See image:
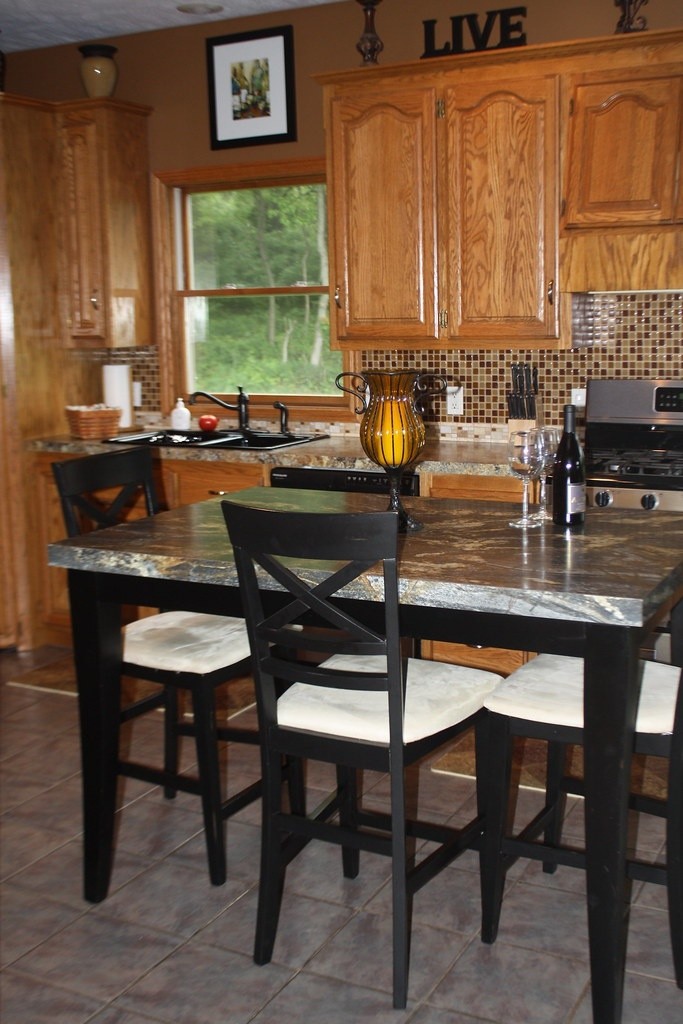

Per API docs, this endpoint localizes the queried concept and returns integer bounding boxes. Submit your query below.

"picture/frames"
[202,23,297,150]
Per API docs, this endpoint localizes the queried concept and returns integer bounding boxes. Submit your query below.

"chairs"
[220,500,507,1010]
[481,563,682,1024]
[271,462,420,496]
[50,445,261,904]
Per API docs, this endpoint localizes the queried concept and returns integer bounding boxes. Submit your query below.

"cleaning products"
[171,398,191,430]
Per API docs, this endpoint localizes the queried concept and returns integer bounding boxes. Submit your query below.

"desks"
[47,476,683,1023]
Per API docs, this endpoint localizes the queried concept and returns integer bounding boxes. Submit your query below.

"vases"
[80,44,120,101]
[356,2,383,67]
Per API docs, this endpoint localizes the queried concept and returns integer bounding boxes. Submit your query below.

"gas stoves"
[585,379,683,511]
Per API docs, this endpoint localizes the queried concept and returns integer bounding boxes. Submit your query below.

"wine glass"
[528,427,558,520]
[508,432,546,528]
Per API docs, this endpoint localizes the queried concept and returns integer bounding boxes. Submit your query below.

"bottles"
[170,398,191,442]
[553,405,585,526]
[78,44,119,97]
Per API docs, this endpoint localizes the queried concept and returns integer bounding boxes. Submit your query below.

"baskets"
[65,405,122,439]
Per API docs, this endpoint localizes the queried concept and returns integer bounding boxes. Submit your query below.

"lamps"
[334,365,462,536]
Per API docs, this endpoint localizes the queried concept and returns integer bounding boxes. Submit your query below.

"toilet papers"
[104,365,131,428]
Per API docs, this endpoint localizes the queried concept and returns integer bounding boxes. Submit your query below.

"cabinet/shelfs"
[51,102,154,353]
[311,29,683,349]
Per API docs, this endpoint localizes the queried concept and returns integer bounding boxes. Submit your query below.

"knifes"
[505,364,538,420]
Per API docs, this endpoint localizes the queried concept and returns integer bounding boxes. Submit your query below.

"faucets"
[273,401,288,433]
[190,386,251,430]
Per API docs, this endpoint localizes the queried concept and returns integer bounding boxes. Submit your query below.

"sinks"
[101,430,230,448]
[208,437,310,450]
